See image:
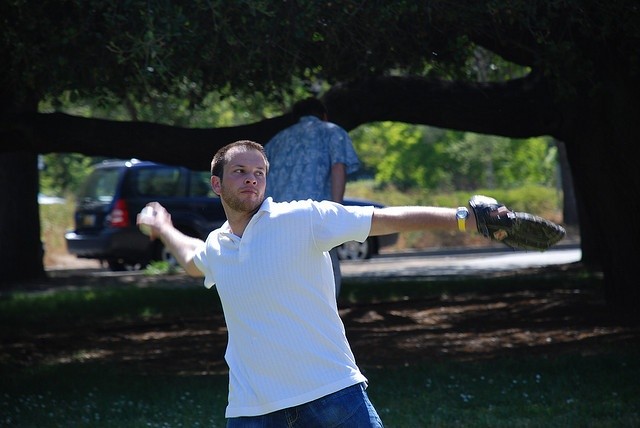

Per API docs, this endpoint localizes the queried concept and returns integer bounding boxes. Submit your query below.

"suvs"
[63,160,400,273]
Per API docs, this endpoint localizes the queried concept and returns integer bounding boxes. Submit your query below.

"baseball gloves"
[471,195,567,254]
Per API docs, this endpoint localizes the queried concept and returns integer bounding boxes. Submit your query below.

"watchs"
[456,206,469,232]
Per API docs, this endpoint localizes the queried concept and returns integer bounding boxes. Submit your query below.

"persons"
[137,140,567,428]
[263,97,360,303]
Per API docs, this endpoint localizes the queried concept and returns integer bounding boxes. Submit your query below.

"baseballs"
[137,204,158,236]
